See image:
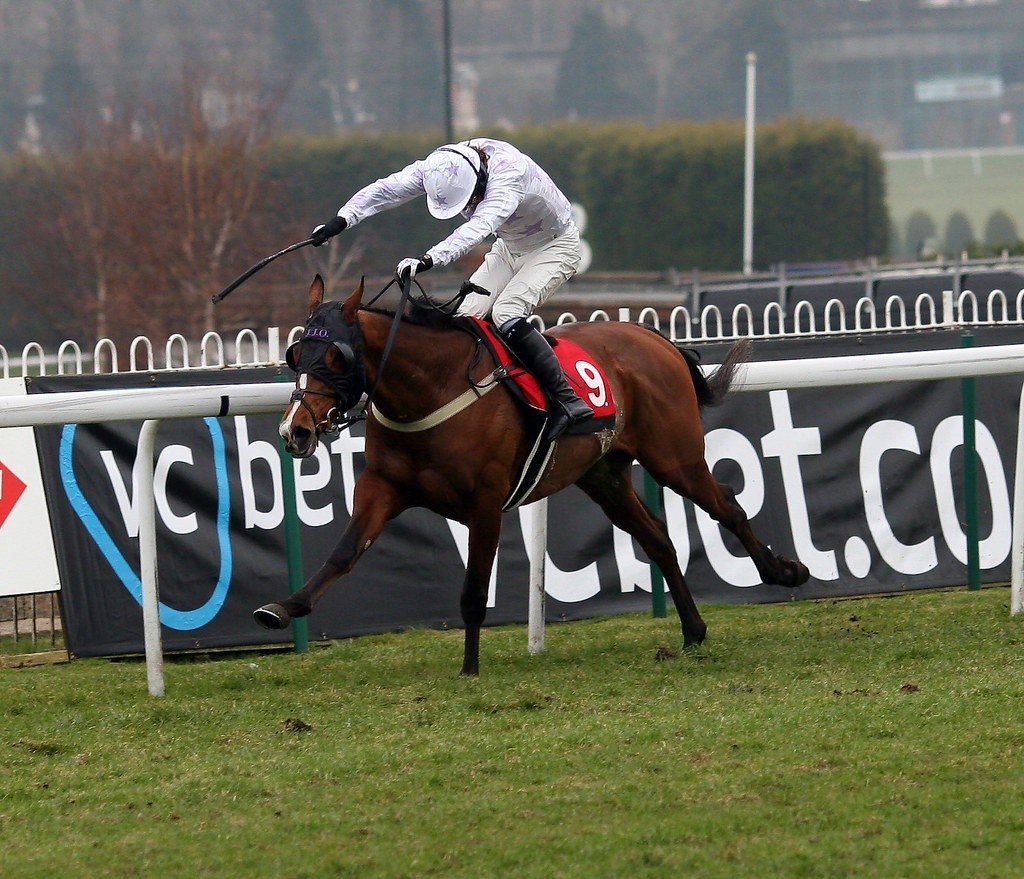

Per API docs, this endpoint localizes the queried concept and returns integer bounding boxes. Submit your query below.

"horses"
[255,272,810,674]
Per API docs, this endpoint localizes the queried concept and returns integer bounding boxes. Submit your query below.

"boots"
[512,328,594,440]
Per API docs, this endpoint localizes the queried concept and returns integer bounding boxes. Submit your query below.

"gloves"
[396,253,433,281]
[309,216,347,247]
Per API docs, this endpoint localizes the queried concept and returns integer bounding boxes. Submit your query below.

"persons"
[310,138,595,441]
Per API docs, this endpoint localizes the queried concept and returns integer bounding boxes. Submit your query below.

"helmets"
[422,143,481,219]
